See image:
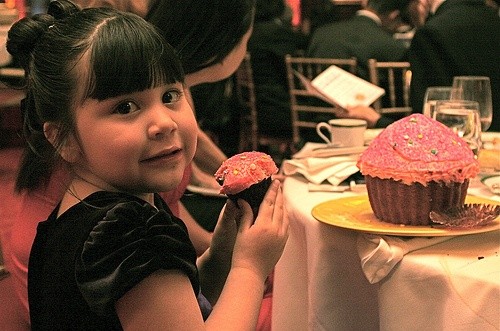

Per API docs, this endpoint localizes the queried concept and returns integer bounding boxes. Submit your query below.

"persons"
[246,0,310,138]
[141,0,287,260]
[5,6,291,330]
[307,0,409,144]
[332,0,500,135]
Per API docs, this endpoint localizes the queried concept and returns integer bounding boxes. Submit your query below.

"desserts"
[357,113,479,225]
[214,151,279,208]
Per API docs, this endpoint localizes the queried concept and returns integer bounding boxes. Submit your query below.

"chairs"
[369,58,413,117]
[285,54,356,146]
[235,52,290,164]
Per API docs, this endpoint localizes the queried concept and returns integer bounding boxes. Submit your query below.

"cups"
[422,87,464,121]
[435,99,482,160]
[316,118,368,148]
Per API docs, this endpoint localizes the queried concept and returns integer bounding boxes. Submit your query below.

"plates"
[310,193,500,236]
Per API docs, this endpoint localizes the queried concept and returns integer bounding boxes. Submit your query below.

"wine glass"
[449,76,493,159]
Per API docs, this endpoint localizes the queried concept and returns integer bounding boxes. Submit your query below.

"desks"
[270,132,500,330]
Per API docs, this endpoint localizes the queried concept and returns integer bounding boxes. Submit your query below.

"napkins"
[357,233,454,286]
[280,157,361,186]
